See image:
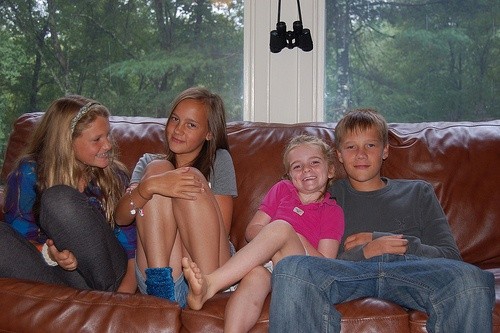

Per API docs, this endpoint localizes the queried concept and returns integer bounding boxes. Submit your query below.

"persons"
[269,107,496,333]
[181,135,345,333]
[0,94,138,294]
[114,85,240,308]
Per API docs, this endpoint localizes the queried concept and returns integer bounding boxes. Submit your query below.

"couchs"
[0,112,500,333]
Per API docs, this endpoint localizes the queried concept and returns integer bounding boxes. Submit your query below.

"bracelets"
[126,190,145,216]
[41,242,60,267]
[136,182,153,201]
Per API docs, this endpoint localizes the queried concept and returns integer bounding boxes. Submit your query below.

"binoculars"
[269,21,314,53]
